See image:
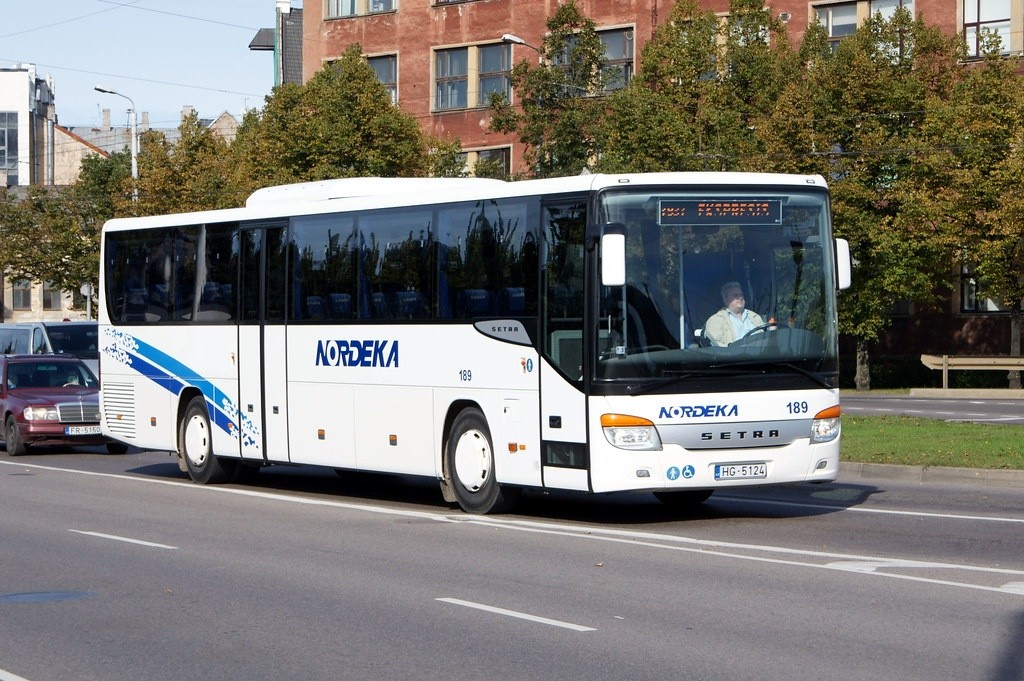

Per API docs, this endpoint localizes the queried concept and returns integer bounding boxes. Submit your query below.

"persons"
[704,281,776,347]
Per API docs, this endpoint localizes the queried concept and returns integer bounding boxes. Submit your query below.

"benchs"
[921,353,1024,388]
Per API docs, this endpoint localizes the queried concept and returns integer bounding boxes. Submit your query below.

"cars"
[0,355,129,457]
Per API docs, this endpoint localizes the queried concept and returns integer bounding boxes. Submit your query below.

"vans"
[0,323,103,387]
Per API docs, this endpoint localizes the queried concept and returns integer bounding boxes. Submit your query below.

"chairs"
[115,281,526,321]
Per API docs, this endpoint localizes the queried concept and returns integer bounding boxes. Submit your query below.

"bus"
[98,179,853,515]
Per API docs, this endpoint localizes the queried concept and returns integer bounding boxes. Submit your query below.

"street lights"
[91,85,141,217]
[501,34,543,176]
